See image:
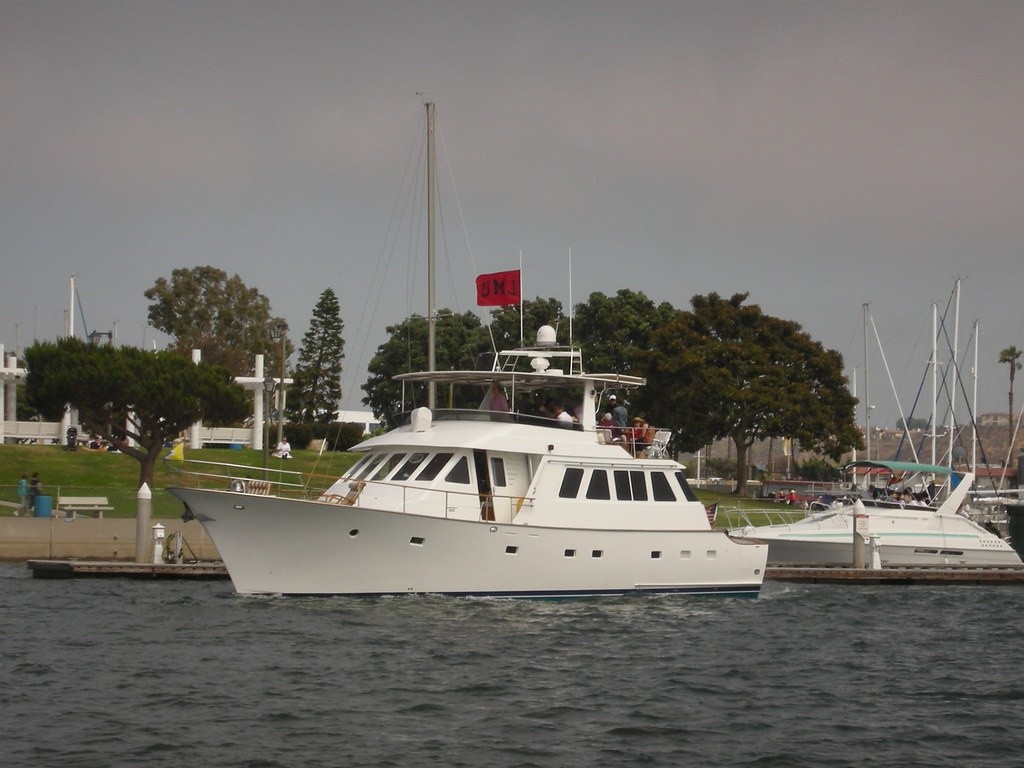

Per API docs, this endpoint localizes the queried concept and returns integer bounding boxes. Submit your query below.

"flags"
[704,502,719,524]
[476,270,521,307]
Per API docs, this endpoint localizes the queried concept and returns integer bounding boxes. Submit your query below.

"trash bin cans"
[33,493,52,517]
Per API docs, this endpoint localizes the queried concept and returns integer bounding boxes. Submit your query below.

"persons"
[14,472,45,516]
[490,381,656,460]
[271,437,293,458]
[84,435,127,453]
[770,480,1001,538]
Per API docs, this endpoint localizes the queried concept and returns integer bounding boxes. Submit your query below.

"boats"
[161,92,770,595]
[722,458,1024,570]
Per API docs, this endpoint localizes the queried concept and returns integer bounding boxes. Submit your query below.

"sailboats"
[813,277,1024,538]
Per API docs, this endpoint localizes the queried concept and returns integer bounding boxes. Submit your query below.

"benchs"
[59,496,114,519]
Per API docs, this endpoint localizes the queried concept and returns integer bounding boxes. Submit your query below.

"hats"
[609,394,617,401]
[790,490,796,493]
[633,417,644,422]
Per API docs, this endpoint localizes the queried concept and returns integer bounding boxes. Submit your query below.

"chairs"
[646,432,672,459]
